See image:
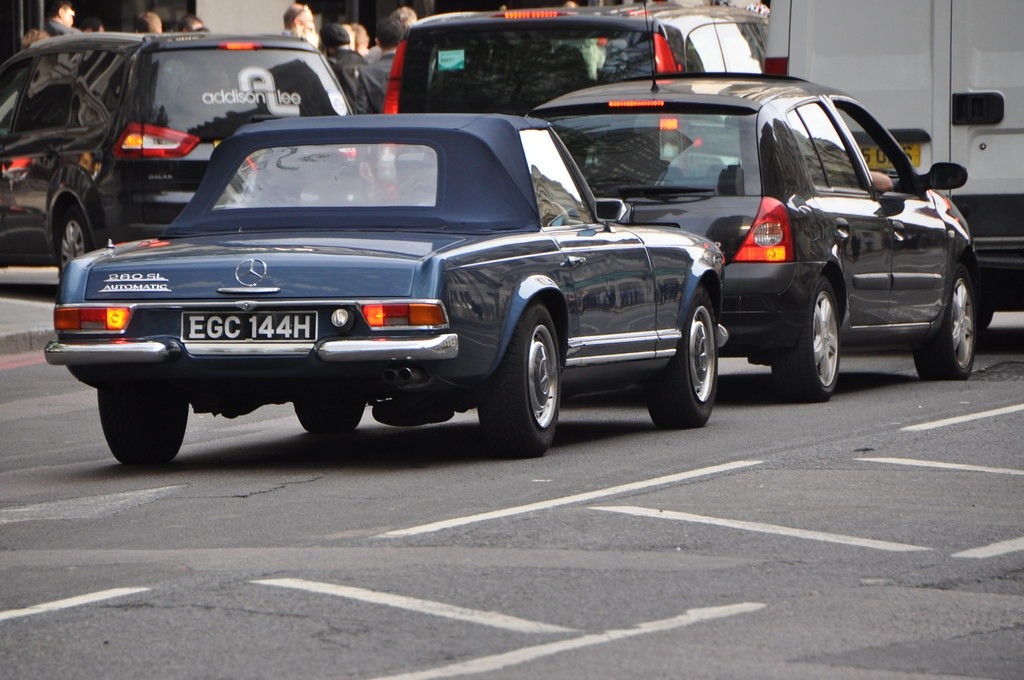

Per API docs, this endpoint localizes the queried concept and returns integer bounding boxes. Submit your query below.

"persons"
[869,171,892,192]
[281,2,418,115]
[20,0,210,49]
[544,0,606,83]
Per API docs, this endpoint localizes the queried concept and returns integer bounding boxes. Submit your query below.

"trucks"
[764,1,1024,325]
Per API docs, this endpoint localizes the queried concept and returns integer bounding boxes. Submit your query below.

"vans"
[385,1,769,157]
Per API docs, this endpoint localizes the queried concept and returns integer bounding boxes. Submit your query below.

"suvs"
[0,31,367,284]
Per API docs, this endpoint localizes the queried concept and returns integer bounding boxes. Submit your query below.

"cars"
[520,72,987,403]
[44,112,728,466]
